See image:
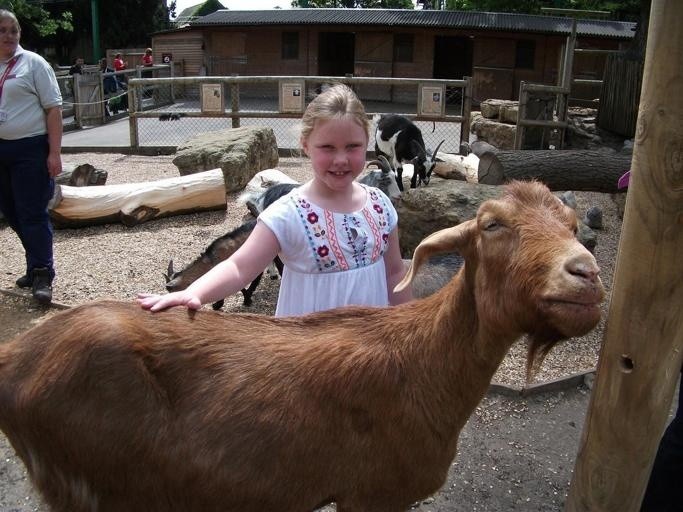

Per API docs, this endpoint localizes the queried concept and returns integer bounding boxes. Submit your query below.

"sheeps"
[162,221,265,311]
[234,169,302,281]
[256,155,403,285]
[372,111,446,186]
[1,176,607,512]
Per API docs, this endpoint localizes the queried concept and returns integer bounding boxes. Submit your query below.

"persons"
[66,58,85,120]
[0,9,64,301]
[97,53,127,116]
[142,48,153,98]
[135,84,414,311]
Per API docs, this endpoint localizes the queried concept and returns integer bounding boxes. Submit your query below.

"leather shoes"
[32,266,53,303]
[16,272,32,287]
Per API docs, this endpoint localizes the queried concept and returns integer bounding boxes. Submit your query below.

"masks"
[47,176,62,215]
